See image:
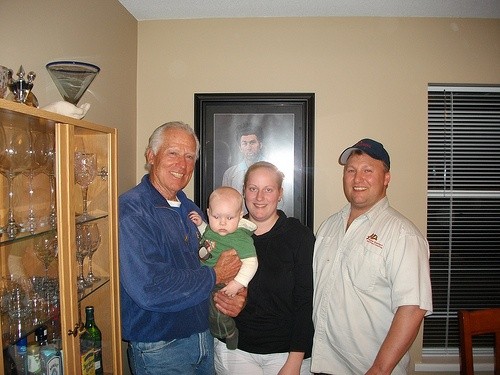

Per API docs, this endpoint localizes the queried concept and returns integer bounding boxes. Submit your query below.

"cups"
[0,274,60,321]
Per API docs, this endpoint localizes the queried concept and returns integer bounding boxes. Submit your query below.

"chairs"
[457,309,500,375]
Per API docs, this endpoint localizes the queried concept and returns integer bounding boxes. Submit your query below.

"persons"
[214,161,316,375]
[188,187,258,350]
[118,121,247,375]
[310,139,433,375]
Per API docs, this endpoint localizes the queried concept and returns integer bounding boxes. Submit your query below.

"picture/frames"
[194,93,315,232]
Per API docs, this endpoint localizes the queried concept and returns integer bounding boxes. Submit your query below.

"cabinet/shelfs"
[0,98,123,375]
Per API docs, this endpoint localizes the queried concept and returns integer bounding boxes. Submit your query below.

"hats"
[338,138,391,166]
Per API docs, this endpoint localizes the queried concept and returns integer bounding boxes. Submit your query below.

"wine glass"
[75,224,93,292]
[46,60,101,106]
[20,129,50,231]
[0,122,31,234]
[43,132,57,226]
[84,223,101,282]
[34,232,58,276]
[74,153,97,221]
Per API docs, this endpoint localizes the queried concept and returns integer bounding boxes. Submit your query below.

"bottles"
[4,320,64,375]
[85,306,103,375]
